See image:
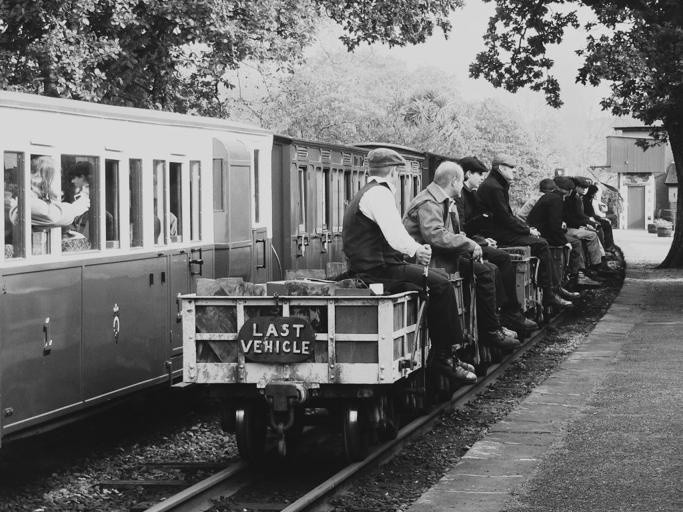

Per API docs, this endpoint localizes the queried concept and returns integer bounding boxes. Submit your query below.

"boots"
[428,346,477,384]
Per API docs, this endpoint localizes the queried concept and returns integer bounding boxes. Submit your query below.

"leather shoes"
[571,271,600,288]
[503,311,538,331]
[542,287,580,307]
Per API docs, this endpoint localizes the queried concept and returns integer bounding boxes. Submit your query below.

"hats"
[460,157,488,173]
[555,176,590,191]
[368,148,406,167]
[493,154,517,168]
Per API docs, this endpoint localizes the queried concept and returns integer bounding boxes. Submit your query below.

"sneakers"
[482,325,520,345]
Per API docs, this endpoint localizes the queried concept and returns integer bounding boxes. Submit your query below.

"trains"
[0,91,461,451]
[163,220,599,466]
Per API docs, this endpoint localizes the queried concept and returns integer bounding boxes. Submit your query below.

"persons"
[478,158,580,308]
[340,146,478,386]
[516,177,617,289]
[455,158,539,332]
[398,159,521,347]
[4,156,177,244]
